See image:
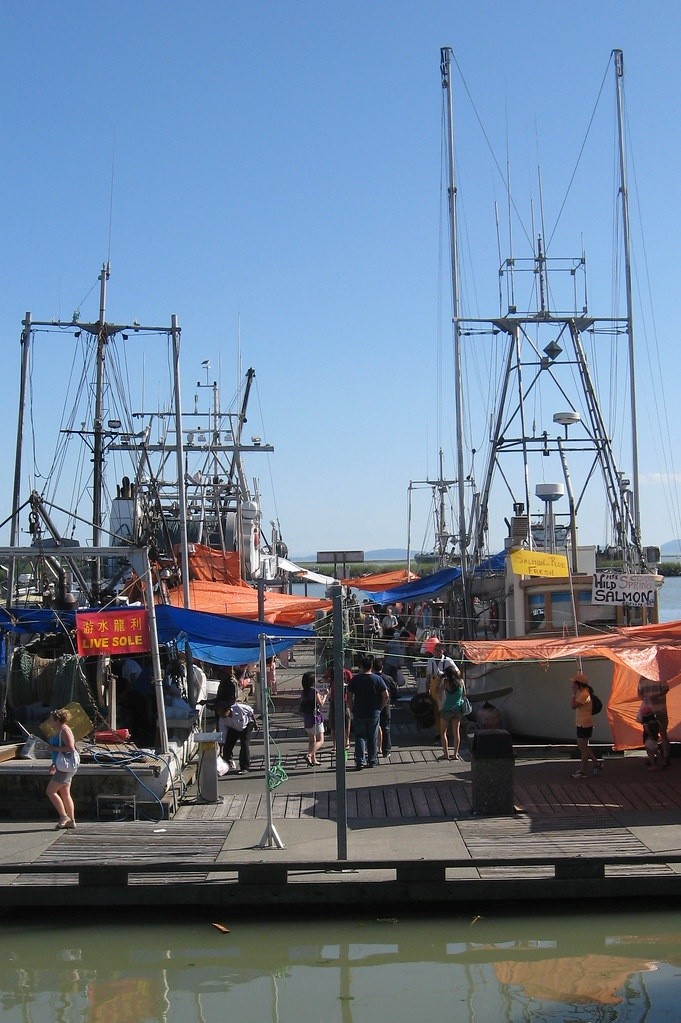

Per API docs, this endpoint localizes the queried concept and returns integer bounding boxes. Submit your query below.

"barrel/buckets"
[409,693,435,729]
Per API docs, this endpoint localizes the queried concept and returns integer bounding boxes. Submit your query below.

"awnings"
[0,543,509,673]
[460,621,681,752]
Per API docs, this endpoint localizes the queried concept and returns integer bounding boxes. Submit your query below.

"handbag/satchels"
[461,699,472,715]
[396,669,406,687]
[55,746,81,773]
[591,694,603,715]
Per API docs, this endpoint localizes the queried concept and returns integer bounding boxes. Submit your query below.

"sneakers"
[592,761,605,777]
[568,767,592,779]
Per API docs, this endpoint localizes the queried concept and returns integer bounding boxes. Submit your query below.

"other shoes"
[303,755,314,768]
[68,819,77,829]
[56,815,71,829]
[314,760,323,766]
[438,754,449,760]
[332,745,391,758]
[368,762,376,768]
[449,755,459,760]
[356,765,364,770]
[241,769,248,775]
[647,758,670,770]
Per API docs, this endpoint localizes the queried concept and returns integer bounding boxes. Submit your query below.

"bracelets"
[571,694,576,696]
[572,691,576,694]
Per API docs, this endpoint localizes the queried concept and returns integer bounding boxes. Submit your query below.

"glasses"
[50,710,57,714]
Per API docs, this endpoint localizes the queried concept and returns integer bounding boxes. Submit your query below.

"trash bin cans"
[470,729,518,818]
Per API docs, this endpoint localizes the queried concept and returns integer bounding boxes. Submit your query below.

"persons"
[635,676,671,771]
[350,592,498,760]
[299,671,330,767]
[568,675,605,779]
[117,658,154,747]
[321,658,398,770]
[199,665,258,775]
[45,709,81,829]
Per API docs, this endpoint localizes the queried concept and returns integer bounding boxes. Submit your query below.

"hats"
[440,666,457,676]
[569,674,588,684]
[362,598,368,603]
[640,706,653,716]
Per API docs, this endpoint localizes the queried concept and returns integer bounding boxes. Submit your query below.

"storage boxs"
[41,701,94,744]
[19,734,51,760]
